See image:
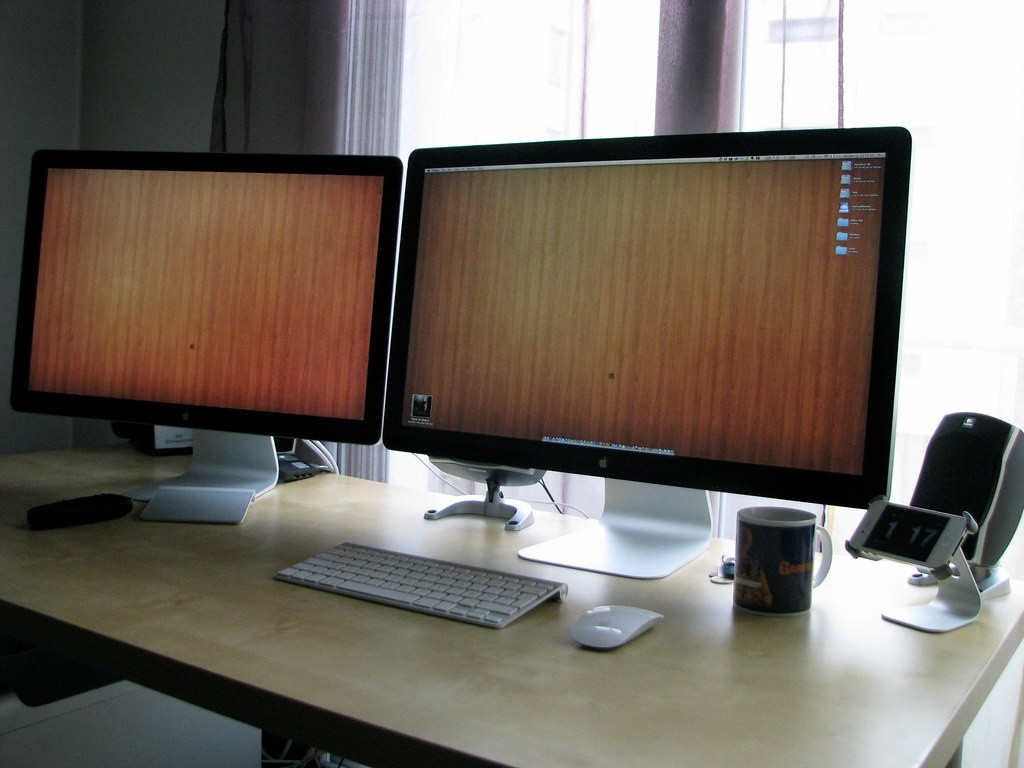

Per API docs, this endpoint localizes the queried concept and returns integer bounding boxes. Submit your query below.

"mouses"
[571,604,664,648]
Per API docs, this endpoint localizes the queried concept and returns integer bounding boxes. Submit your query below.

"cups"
[733,507,831,618]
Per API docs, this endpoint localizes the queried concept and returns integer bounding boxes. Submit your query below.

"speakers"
[911,412,1024,599]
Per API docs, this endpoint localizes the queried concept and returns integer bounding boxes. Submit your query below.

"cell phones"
[849,501,967,566]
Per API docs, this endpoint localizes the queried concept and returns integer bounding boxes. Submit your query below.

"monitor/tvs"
[384,126,912,582]
[9,148,404,523]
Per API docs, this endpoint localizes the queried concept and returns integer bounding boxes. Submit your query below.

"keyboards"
[271,541,569,629]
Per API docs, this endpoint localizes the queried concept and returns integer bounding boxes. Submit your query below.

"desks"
[0,444,1024,768]
